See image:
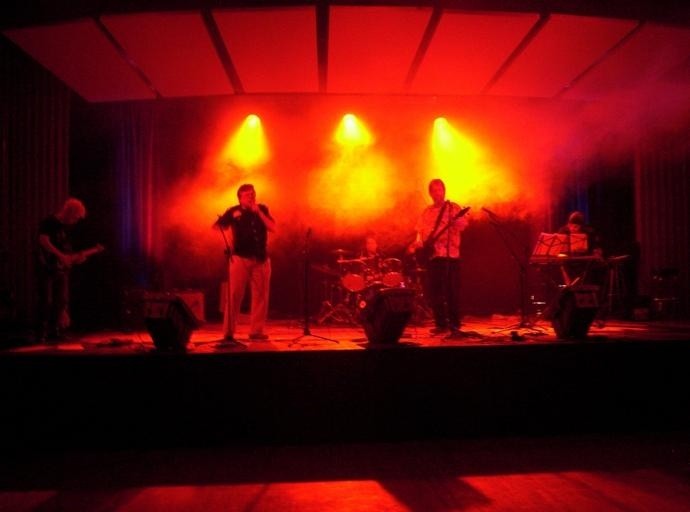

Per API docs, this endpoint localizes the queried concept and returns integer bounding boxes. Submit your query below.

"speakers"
[360,287,416,342]
[143,294,196,351]
[551,285,599,339]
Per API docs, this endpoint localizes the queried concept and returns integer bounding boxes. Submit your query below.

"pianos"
[529,254,605,266]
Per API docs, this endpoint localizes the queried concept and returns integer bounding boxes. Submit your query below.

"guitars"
[415,207,471,265]
[36,239,106,277]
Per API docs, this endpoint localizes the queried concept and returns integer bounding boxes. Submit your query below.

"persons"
[38,199,104,342]
[554,213,608,288]
[213,184,278,344]
[407,180,468,335]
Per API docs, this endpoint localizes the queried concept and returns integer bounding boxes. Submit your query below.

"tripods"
[288,238,340,348]
[196,225,260,349]
[488,217,548,334]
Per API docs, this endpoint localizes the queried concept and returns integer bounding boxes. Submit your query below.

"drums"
[342,259,362,274]
[357,281,393,313]
[381,257,405,284]
[338,273,366,305]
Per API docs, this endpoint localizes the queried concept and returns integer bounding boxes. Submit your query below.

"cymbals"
[311,263,342,277]
[406,266,427,273]
[317,250,359,256]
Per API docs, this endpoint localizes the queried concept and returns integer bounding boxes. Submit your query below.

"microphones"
[482,206,496,217]
